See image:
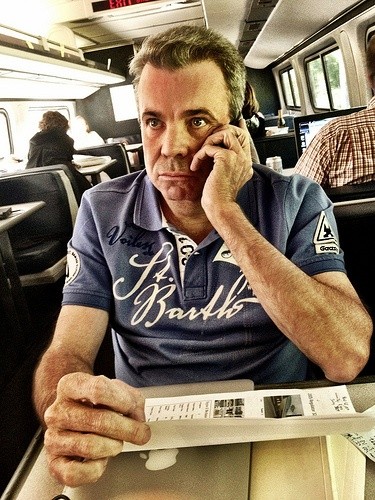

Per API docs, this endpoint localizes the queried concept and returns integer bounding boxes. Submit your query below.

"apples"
[138,447,179,470]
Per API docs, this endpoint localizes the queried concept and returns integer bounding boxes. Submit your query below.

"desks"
[4,383,375,500]
[0,199,46,320]
[126,143,144,167]
[77,157,118,185]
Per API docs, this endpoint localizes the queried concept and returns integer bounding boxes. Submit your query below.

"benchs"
[0,112,375,377]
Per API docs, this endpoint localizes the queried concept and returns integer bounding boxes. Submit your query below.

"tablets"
[59,378,253,500]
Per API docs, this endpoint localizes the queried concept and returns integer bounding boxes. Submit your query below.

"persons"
[24,107,77,177]
[293,34,374,199]
[32,23,374,490]
